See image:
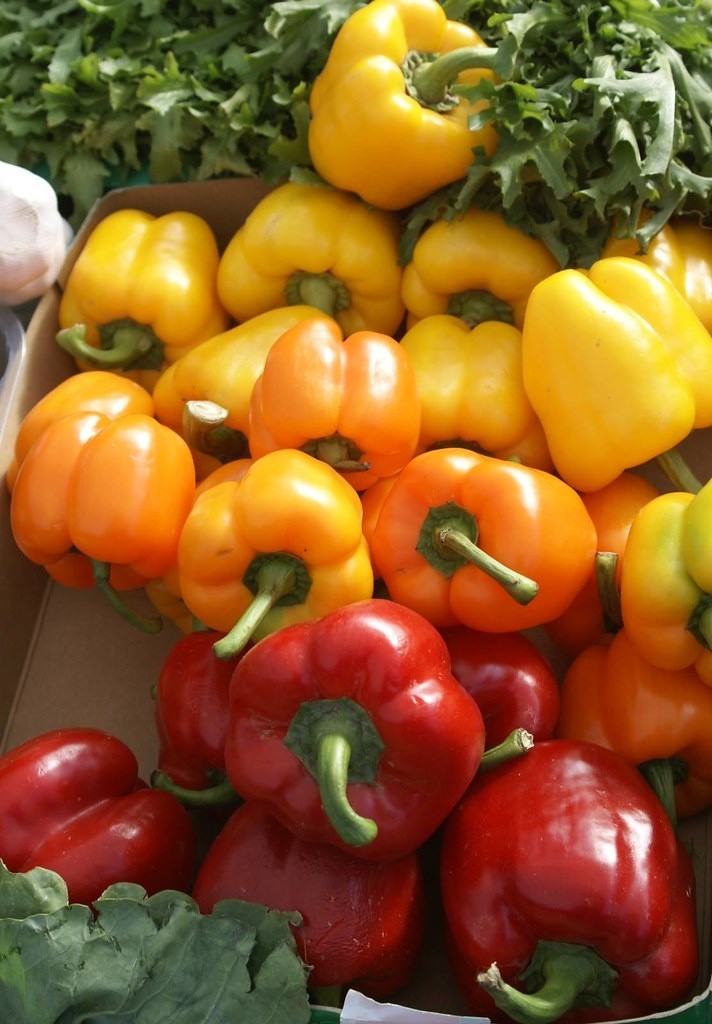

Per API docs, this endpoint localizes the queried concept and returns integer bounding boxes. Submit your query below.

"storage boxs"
[0,184,712,1020]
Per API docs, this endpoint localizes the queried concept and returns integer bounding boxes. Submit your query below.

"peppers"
[1,181,712,1023]
[307,1,507,212]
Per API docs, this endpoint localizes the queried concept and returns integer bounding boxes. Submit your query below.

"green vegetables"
[0,0,712,270]
